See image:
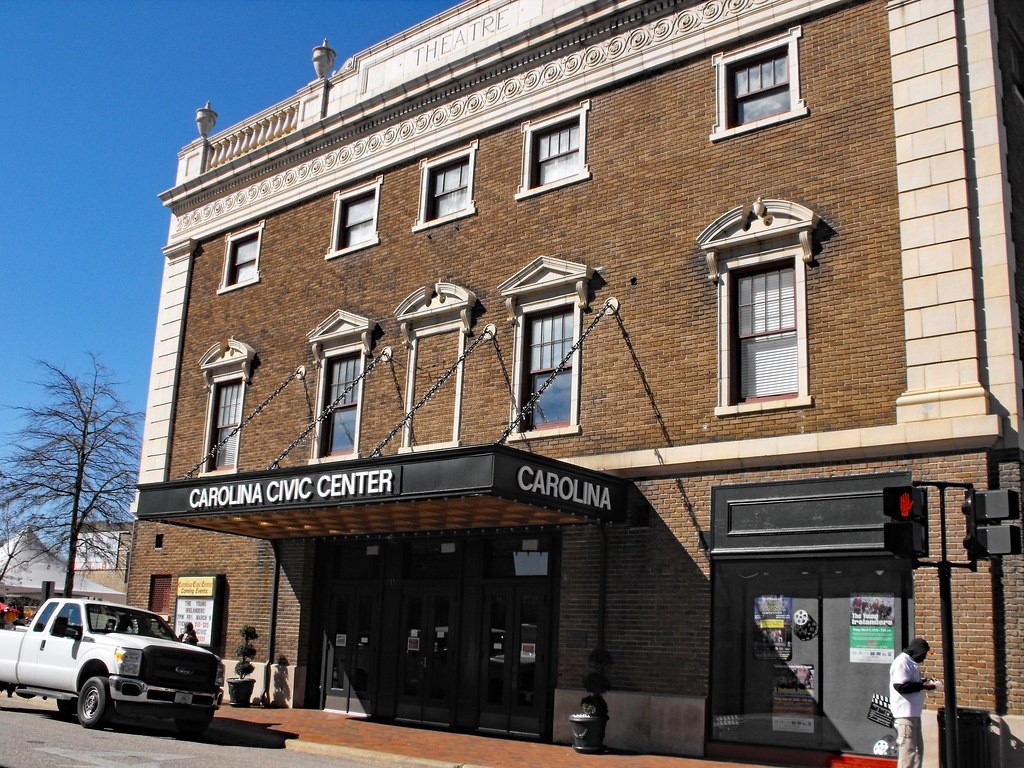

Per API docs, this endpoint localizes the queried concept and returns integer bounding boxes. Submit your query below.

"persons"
[890,638,936,768]
[177,622,199,646]
[852,594,891,620]
[11,597,16,609]
[0,612,7,629]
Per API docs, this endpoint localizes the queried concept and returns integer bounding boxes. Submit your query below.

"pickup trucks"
[0,596,226,738]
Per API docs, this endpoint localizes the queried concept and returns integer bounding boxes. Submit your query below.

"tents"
[0,526,127,595]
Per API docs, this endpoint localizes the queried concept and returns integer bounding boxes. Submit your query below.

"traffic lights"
[882,485,929,560]
[961,490,1023,559]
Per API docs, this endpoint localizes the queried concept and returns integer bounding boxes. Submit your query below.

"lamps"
[753,196,772,225]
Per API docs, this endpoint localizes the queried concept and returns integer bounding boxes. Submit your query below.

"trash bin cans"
[937,707,991,768]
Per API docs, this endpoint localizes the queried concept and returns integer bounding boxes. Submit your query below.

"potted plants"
[226,625,261,707]
[569,646,615,754]
